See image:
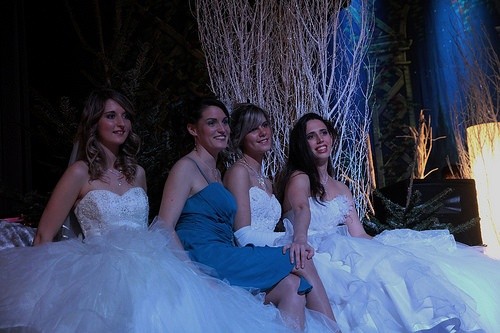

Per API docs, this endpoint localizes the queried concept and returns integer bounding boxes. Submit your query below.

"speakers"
[374,179,485,248]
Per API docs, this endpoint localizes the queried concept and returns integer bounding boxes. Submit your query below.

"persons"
[0,86,303,333]
[273,113,500,333]
[158,97,341,333]
[222,102,403,333]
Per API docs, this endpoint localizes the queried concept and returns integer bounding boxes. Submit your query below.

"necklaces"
[194,148,218,182]
[239,159,266,191]
[320,176,333,194]
[108,168,122,186]
